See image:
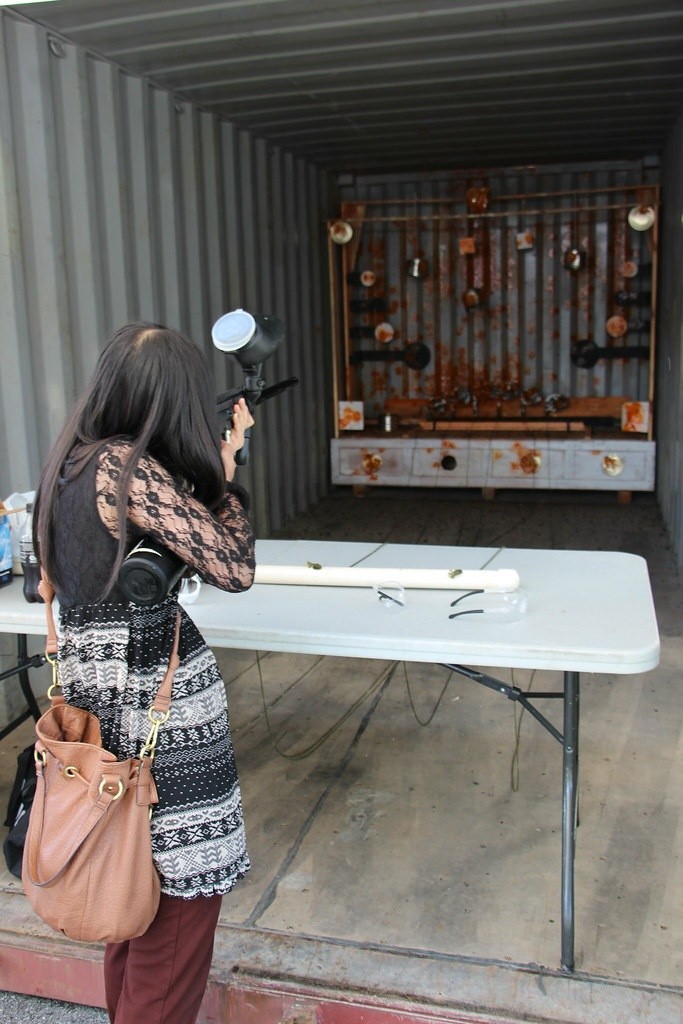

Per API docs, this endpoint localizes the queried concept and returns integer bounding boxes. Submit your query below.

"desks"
[0,537,665,979]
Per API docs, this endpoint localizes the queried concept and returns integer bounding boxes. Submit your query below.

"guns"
[204,308,301,467]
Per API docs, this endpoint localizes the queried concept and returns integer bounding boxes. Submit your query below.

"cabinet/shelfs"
[330,418,658,506]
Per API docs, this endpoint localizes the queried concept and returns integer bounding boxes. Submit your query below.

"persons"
[33,324,257,1024]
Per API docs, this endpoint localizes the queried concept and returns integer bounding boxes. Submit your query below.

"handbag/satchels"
[21,703,162,941]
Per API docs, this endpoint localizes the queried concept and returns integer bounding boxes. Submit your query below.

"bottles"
[17,503,55,603]
